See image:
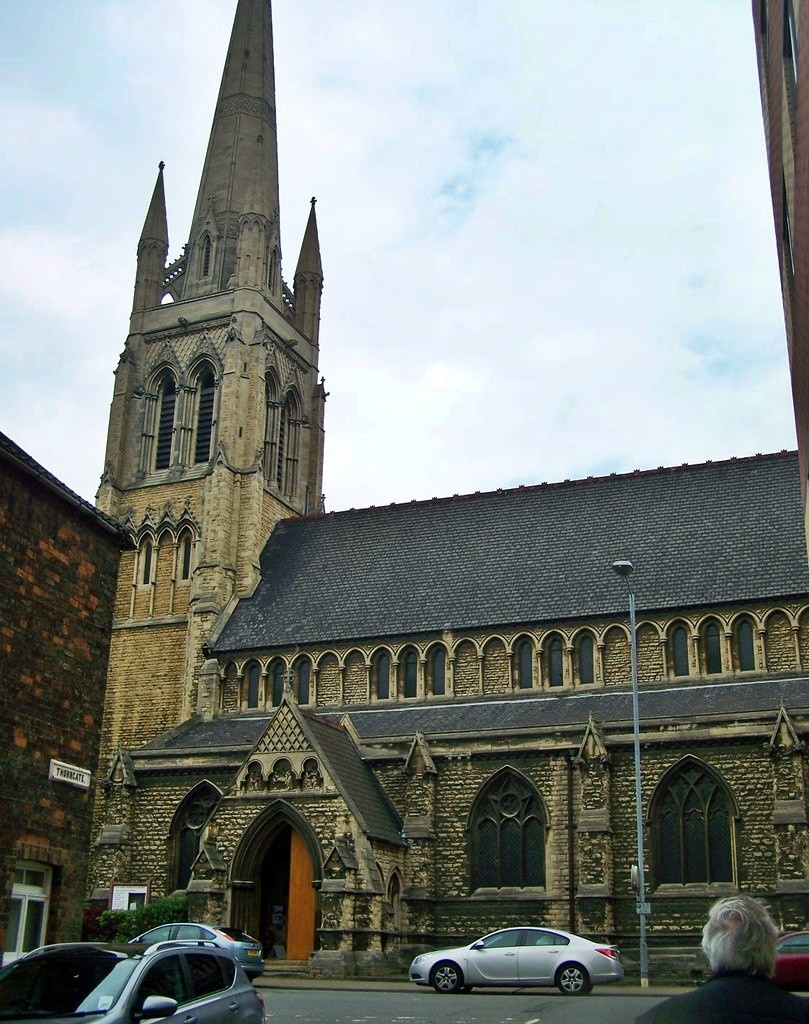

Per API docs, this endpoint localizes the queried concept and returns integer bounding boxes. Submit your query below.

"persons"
[263,924,277,950]
[635,895,809,1024]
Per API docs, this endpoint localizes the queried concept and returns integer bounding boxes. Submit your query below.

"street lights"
[611,558,649,988]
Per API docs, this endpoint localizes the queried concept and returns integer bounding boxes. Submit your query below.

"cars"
[769,930,809,989]
[407,927,625,995]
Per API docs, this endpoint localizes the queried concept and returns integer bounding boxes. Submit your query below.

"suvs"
[127,922,265,984]
[1,939,269,1024]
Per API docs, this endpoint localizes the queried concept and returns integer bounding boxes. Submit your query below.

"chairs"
[536,936,554,944]
